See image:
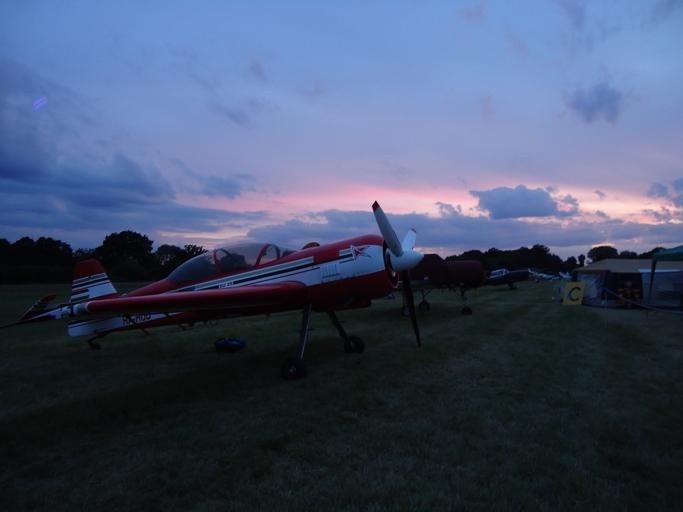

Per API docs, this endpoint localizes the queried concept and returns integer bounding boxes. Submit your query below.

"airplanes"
[0,202,426,378]
[401,255,554,320]
